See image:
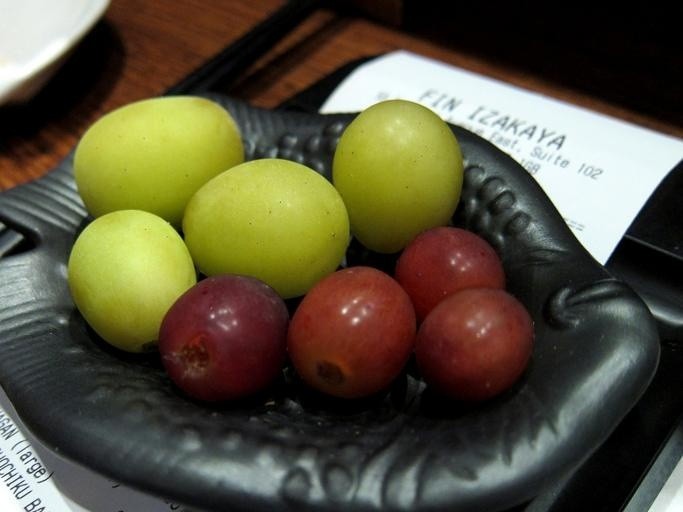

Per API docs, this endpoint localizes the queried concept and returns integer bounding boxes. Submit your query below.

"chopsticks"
[0,0,327,262]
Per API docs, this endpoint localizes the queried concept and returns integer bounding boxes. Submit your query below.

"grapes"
[67,93,533,398]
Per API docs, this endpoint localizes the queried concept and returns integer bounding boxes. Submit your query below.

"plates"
[0,94,660,511]
